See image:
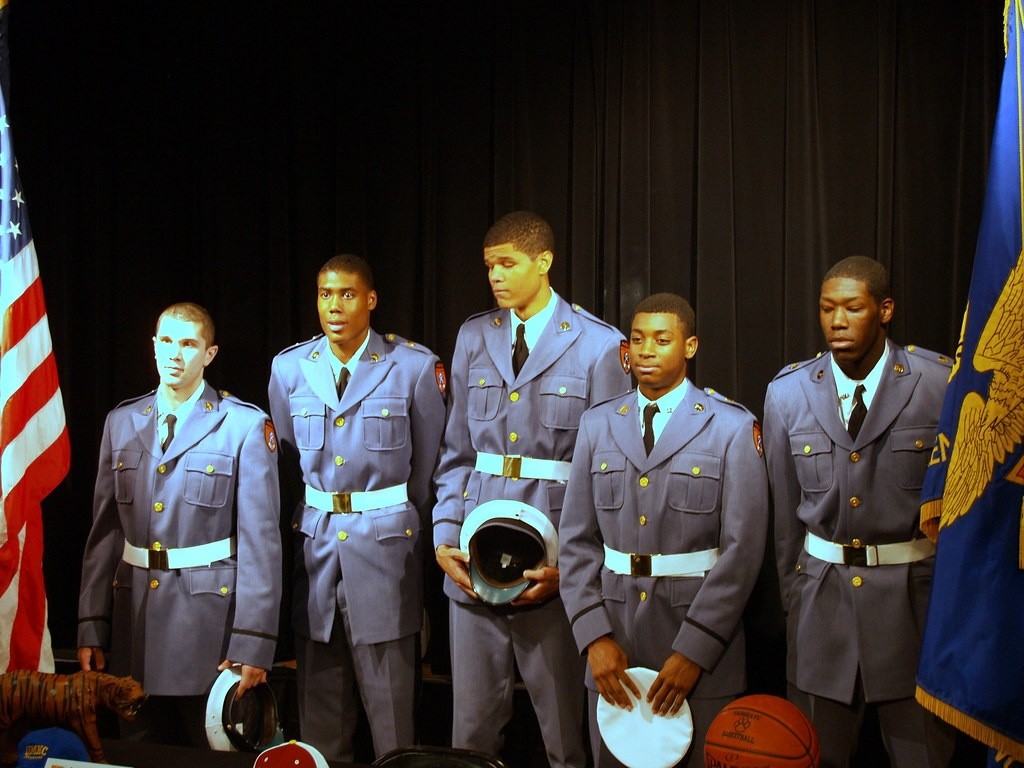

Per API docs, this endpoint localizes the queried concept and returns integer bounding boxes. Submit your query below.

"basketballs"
[703,693,820,768]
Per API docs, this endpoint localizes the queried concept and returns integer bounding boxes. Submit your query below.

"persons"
[76,304,283,753]
[267,254,447,768]
[557,292,769,768]
[762,257,956,768]
[432,212,633,768]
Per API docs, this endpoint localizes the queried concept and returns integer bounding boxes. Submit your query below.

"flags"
[0,94,73,675]
[915,0,1024,768]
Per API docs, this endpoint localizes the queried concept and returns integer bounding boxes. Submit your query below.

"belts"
[120,535,238,570]
[803,527,936,566]
[304,481,409,514]
[603,542,720,576]
[475,451,571,481]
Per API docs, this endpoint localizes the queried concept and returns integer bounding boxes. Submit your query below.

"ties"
[511,325,530,376]
[163,416,173,451]
[336,367,353,399]
[640,405,660,457]
[845,386,870,442]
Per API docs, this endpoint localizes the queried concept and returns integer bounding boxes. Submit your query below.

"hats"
[460,498,561,606]
[252,739,335,767]
[597,667,693,767]
[14,725,91,768]
[205,665,277,752]
